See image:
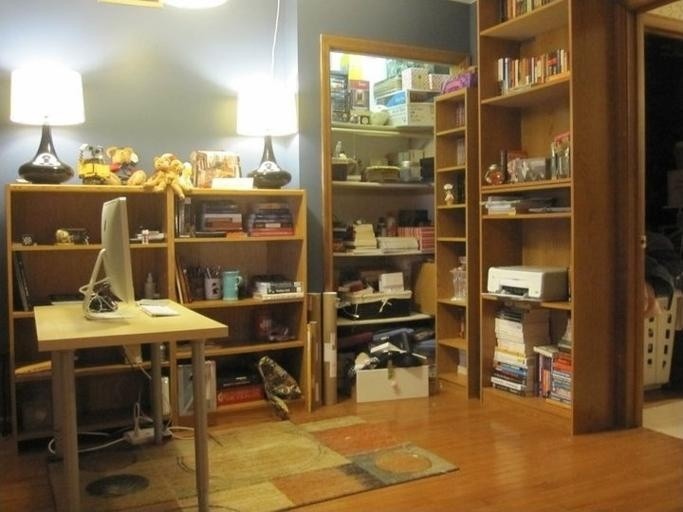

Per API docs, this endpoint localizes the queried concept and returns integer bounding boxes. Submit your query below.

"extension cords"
[125,423,173,445]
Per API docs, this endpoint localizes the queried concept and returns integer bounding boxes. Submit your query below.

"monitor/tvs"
[82,197,136,321]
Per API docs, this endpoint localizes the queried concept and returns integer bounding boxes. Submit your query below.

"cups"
[370,112,390,125]
[204,278,222,301]
[222,271,244,301]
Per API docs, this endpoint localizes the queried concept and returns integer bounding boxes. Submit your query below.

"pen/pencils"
[201,265,220,279]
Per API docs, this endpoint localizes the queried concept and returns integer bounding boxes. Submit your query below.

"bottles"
[399,160,421,181]
[377,217,387,236]
[332,158,349,181]
[387,210,398,236]
[143,272,157,299]
[485,162,506,185]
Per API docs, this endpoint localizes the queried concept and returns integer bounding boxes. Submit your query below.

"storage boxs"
[329,67,451,129]
[350,365,429,403]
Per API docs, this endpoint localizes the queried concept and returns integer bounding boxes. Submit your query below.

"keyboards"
[139,303,178,316]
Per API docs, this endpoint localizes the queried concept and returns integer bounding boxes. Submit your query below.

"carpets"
[46,414,459,511]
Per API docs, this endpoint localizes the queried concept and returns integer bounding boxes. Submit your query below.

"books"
[490,302,572,414]
[174,197,303,306]
[496,48,568,96]
[48,294,83,305]
[13,251,33,311]
[456,100,466,166]
[344,224,421,256]
[482,195,570,215]
[140,305,181,317]
[385,149,424,166]
[496,0,551,25]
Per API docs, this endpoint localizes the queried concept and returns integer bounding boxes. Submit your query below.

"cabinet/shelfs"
[5,185,173,457]
[320,34,471,393]
[476,0,619,436]
[434,88,480,399]
[172,188,312,428]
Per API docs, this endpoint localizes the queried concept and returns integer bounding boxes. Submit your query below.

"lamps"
[236,79,298,189]
[10,66,87,184]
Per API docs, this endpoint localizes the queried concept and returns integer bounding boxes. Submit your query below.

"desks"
[33,298,228,511]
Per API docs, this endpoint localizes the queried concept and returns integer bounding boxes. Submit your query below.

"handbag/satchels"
[255,357,302,399]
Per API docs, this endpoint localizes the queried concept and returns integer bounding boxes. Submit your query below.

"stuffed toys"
[107,146,146,185]
[144,153,193,200]
[78,144,111,184]
